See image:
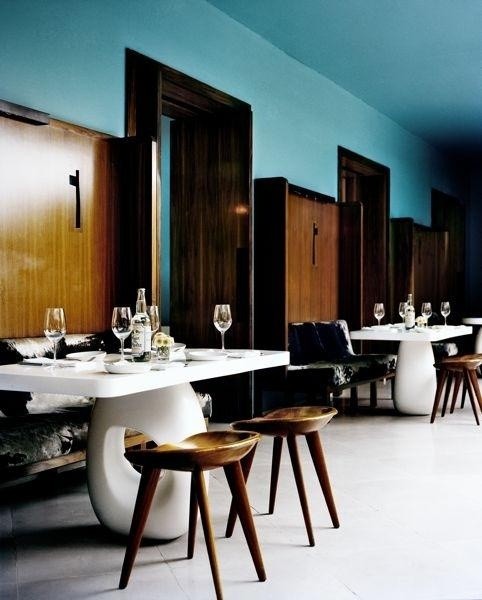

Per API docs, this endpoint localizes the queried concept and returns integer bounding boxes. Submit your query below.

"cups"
[156,336,174,364]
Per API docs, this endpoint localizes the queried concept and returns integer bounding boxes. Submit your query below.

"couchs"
[0,326,213,483]
[289,319,397,417]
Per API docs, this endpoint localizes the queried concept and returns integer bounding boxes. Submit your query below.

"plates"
[152,343,187,352]
[189,351,228,360]
[105,362,151,374]
[65,350,106,362]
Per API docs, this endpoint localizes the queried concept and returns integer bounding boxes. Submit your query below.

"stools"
[119,432,266,600]
[430,353,481,426]
[226,404,338,546]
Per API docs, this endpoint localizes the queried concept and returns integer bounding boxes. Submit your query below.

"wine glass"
[374,303,385,330]
[214,304,234,354]
[441,301,450,327]
[146,306,159,336]
[112,306,133,365]
[44,308,67,366]
[399,303,407,319]
[422,303,432,330]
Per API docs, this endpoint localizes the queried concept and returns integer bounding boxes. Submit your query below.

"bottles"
[132,288,151,362]
[404,294,415,333]
